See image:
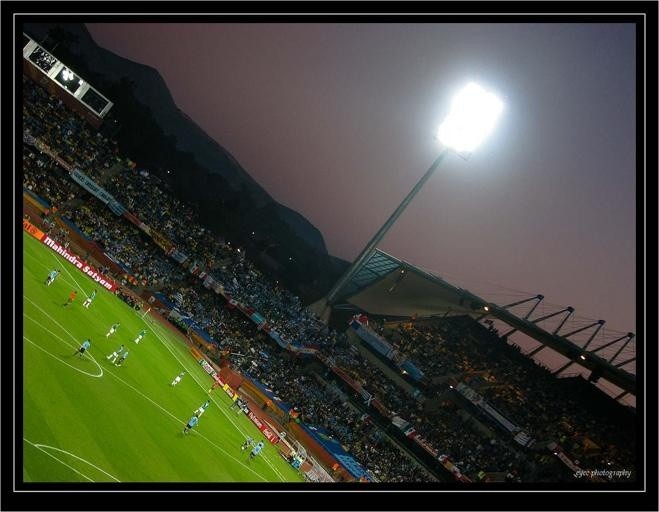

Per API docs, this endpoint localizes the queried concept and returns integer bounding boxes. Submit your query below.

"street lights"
[305,82,506,331]
